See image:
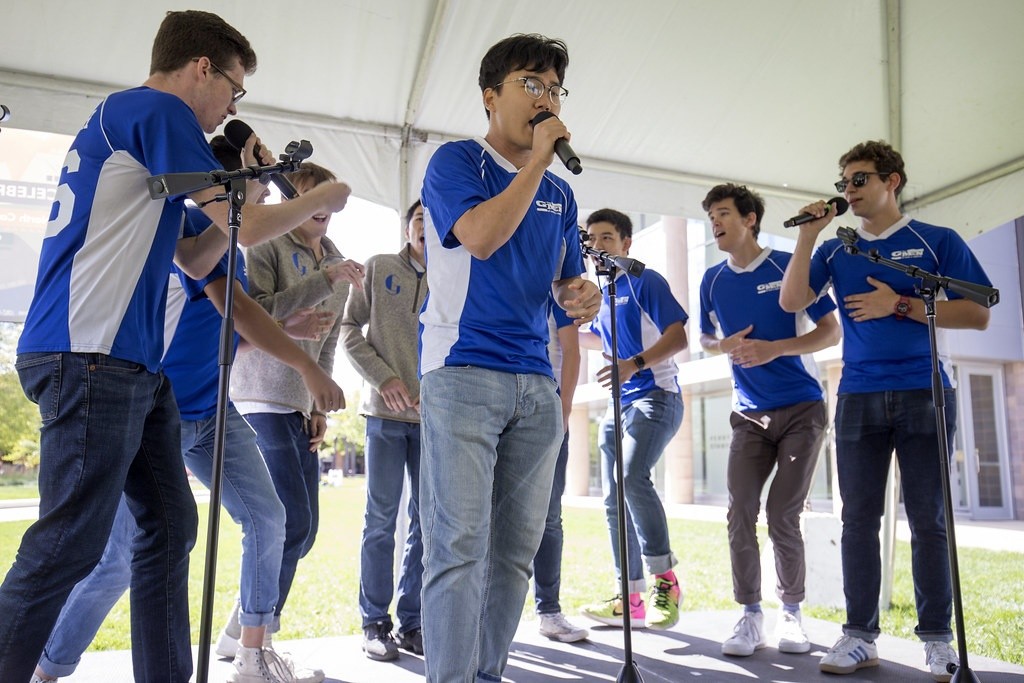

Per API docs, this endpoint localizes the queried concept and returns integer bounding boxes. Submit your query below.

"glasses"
[492,77,569,106]
[835,172,890,193]
[191,57,247,103]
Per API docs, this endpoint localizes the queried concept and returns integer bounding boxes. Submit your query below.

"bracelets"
[277,321,283,327]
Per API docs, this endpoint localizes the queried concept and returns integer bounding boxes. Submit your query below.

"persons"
[699,184,841,655]
[419,34,602,682]
[778,141,993,683]
[578,209,688,632]
[529,294,588,642]
[0,9,352,683]
[214,164,365,658]
[340,201,424,660]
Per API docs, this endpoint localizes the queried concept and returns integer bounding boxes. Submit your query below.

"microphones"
[784,197,850,228]
[531,109,583,176]
[224,119,299,200]
[578,225,590,241]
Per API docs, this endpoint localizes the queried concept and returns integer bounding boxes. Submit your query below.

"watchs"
[895,296,912,320]
[633,355,645,371]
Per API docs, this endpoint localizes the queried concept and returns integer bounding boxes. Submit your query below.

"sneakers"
[538,613,589,642]
[393,627,424,655]
[777,605,810,653]
[924,642,959,682]
[362,621,400,660]
[580,594,645,628]
[722,612,766,655]
[645,573,684,631]
[819,635,880,673]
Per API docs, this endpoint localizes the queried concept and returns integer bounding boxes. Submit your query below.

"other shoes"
[226,640,294,683]
[267,654,325,683]
[214,629,291,657]
[30,674,58,683]
